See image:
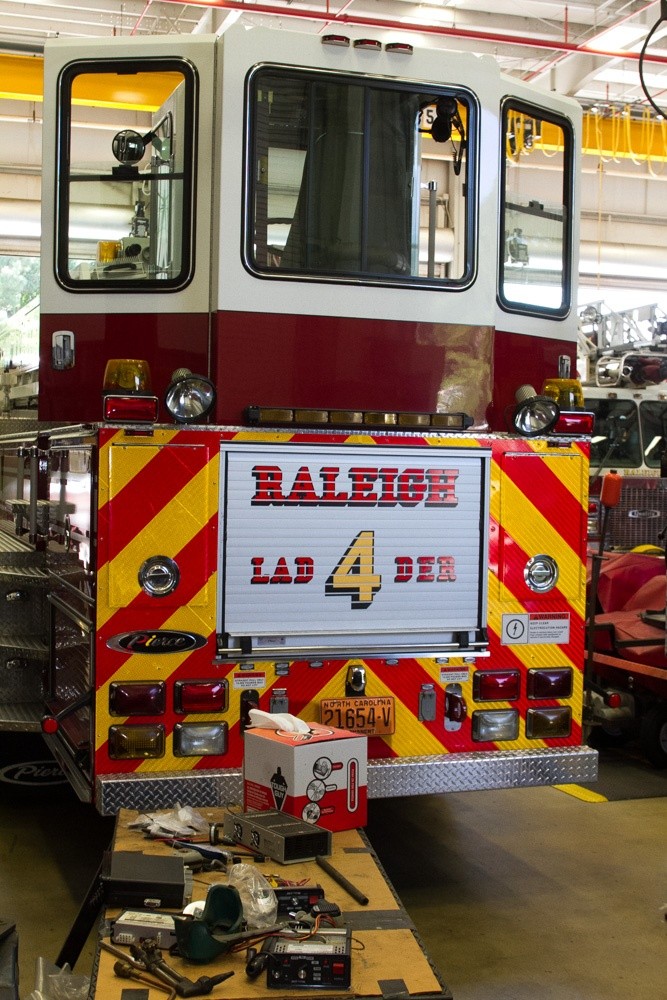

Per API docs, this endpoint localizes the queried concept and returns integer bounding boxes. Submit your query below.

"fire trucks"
[0,1,604,822]
[573,300,667,775]
